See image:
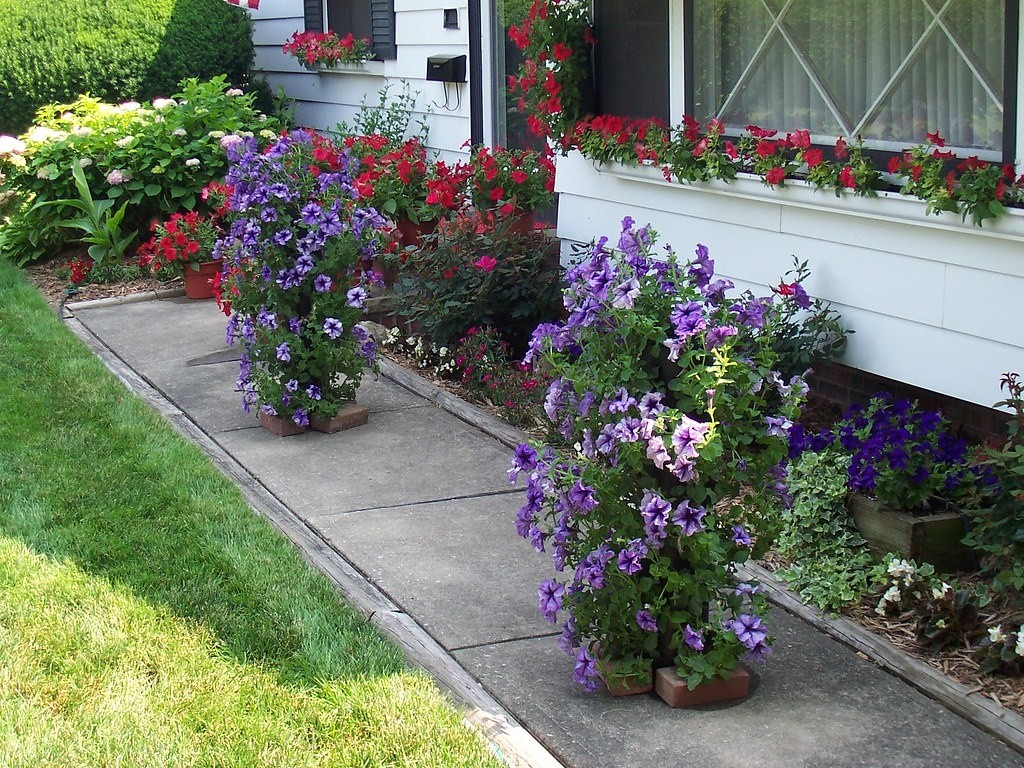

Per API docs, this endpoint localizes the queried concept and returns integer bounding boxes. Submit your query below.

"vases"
[395,216,440,254]
[499,207,534,238]
[844,488,978,572]
[181,258,224,300]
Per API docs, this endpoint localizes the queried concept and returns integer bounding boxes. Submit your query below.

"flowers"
[474,142,556,215]
[134,210,220,275]
[789,390,1003,510]
[353,136,474,220]
